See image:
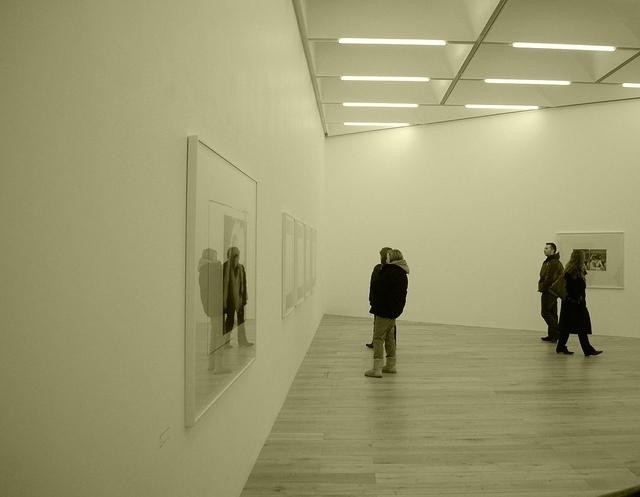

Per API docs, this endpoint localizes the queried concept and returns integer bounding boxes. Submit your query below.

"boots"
[364,358,384,378]
[382,356,397,373]
[208,353,232,375]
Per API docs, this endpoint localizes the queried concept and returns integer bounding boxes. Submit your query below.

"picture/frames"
[184,133,257,429]
[281,211,318,321]
[556,231,626,290]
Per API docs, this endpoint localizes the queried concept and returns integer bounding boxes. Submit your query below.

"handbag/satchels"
[546,273,568,300]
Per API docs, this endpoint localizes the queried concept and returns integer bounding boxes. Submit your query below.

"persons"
[222,245,253,349]
[533,240,563,348]
[554,249,604,357]
[365,247,396,358]
[584,251,603,270]
[363,249,409,378]
[197,245,233,376]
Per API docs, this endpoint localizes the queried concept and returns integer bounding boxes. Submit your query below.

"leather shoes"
[584,346,603,356]
[225,342,253,348]
[539,336,557,343]
[365,342,373,347]
[556,344,574,354]
